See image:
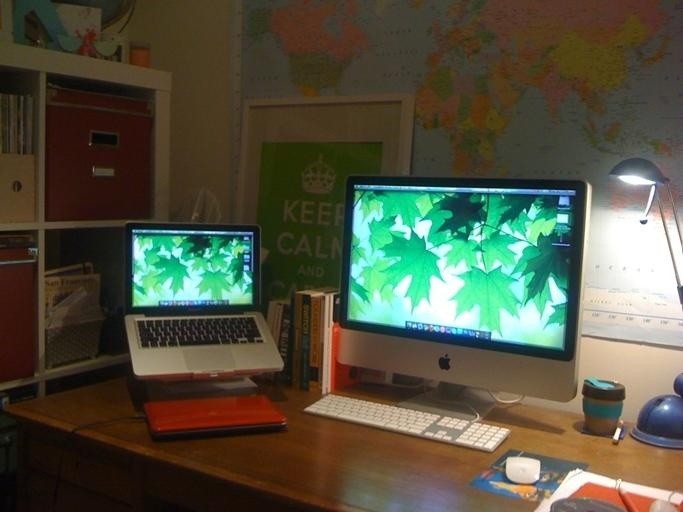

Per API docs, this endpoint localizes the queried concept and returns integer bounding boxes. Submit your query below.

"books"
[45,262,105,330]
[256,286,361,395]
[0,93,34,154]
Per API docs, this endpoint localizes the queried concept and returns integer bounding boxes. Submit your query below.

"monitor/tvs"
[335,174,593,420]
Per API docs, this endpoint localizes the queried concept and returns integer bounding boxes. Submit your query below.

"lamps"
[609,158,683,449]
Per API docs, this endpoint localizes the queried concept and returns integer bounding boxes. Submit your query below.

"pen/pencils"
[612,419,624,444]
[614,479,639,512]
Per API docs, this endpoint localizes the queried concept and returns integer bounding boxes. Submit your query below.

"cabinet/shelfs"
[0,40,172,477]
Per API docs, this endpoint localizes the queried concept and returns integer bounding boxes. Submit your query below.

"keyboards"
[303,394,512,452]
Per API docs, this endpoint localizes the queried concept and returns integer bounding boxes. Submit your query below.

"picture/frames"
[233,93,416,322]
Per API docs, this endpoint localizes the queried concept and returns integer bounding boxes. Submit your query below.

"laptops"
[122,220,285,381]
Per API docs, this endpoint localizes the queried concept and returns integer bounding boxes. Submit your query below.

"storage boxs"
[46,83,153,221]
[0,235,39,384]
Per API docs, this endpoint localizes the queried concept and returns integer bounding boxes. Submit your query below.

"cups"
[581,380,626,434]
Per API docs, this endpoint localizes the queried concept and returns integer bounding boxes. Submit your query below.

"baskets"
[44,316,105,368]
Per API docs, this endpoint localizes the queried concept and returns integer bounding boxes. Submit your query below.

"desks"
[2,373,683,512]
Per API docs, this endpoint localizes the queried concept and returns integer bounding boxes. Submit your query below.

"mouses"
[505,457,542,486]
